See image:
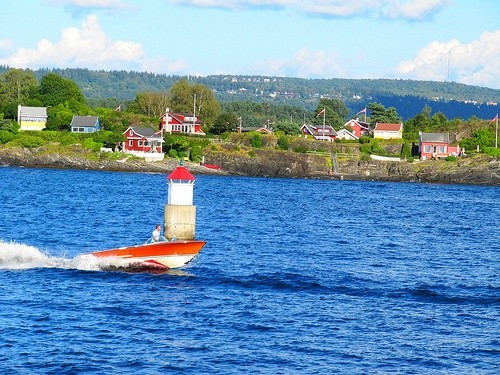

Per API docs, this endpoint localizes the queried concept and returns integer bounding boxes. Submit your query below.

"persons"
[150,225,161,243]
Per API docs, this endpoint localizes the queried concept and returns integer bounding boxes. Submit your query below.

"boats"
[92,241,205,274]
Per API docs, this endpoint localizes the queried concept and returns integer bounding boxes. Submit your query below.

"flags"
[490,114,498,123]
[318,110,324,115]
[358,109,366,114]
